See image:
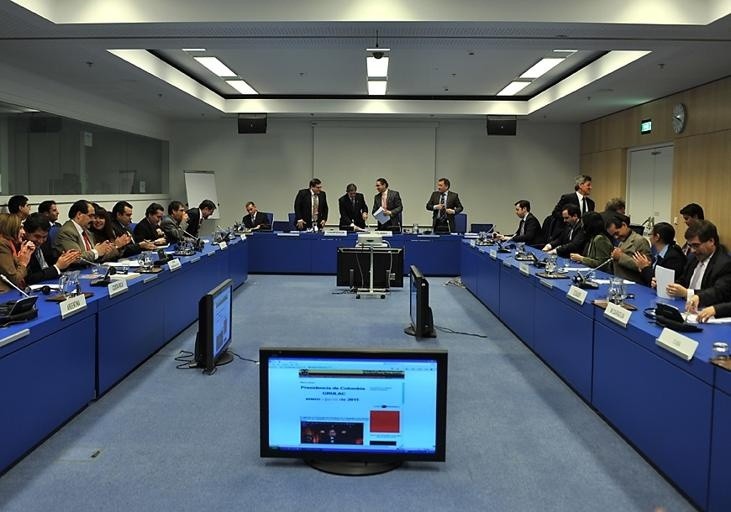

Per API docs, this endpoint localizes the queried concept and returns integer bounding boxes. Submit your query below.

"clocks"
[670,102,686,134]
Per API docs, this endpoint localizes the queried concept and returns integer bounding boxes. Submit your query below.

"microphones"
[0,225,243,327]
[352,219,451,235]
[475,224,704,333]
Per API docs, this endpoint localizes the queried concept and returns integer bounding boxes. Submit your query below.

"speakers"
[238,118,266,133]
[487,120,516,136]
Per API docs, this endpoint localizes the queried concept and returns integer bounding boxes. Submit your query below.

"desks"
[592,290,730,511]
[459,235,624,404]
[1,290,97,474]
[33,233,247,400]
[236,223,464,277]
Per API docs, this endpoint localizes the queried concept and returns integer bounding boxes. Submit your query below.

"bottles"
[412,222,419,235]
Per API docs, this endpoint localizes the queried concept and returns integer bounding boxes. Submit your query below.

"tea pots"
[58,269,82,302]
[545,251,558,276]
[137,249,152,270]
[608,274,626,308]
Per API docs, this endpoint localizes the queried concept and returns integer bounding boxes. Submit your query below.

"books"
[373,206,390,225]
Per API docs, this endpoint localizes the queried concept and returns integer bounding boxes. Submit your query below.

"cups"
[122,262,129,272]
[90,262,100,275]
[713,341,731,364]
[563,257,570,268]
[590,269,597,281]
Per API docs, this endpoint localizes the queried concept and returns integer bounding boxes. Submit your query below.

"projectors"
[358,234,383,243]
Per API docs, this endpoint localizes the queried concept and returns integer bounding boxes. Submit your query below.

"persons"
[370,177,403,234]
[241,201,269,228]
[337,183,368,231]
[0,193,216,291]
[291,178,327,230]
[491,199,541,245]
[549,172,731,324]
[425,178,463,232]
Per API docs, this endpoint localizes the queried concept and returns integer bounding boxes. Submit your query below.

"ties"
[515,219,525,236]
[81,231,92,251]
[36,249,45,269]
[568,225,575,242]
[690,262,705,292]
[312,193,319,224]
[582,197,587,215]
[439,193,447,225]
[252,216,255,224]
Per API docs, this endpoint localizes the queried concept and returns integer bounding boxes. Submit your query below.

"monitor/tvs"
[189,279,233,371]
[259,346,448,475]
[337,246,403,292]
[404,264,437,338]
